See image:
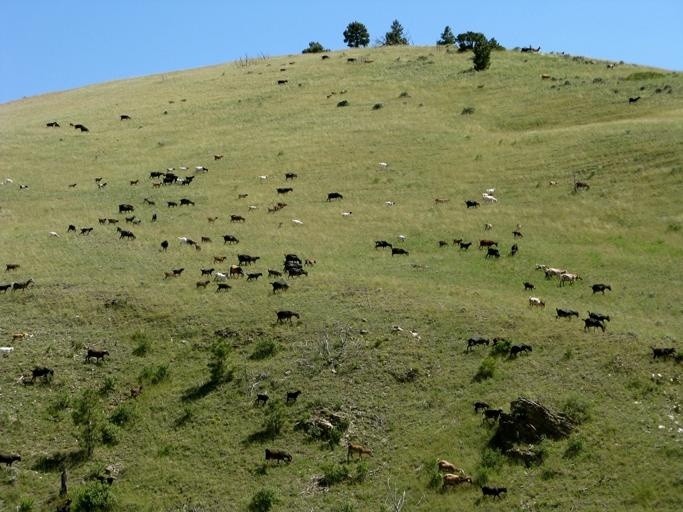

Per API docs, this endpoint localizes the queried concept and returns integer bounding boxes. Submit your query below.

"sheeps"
[0,455,21,466]
[148,165,207,188]
[327,192,353,218]
[437,237,474,252]
[92,175,110,189]
[176,212,246,250]
[131,178,139,186]
[83,346,110,366]
[510,243,523,258]
[463,335,535,359]
[116,228,139,239]
[574,181,589,197]
[214,155,224,160]
[589,280,611,296]
[534,262,584,287]
[478,238,504,260]
[432,197,450,206]
[485,221,496,231]
[527,297,548,306]
[467,198,482,209]
[0,278,33,296]
[260,170,298,195]
[99,216,119,224]
[473,400,504,425]
[196,254,315,293]
[481,185,498,202]
[143,196,197,220]
[350,318,423,348]
[274,307,300,324]
[158,240,175,250]
[67,179,77,190]
[653,348,676,362]
[164,266,183,280]
[245,202,305,226]
[106,298,120,308]
[519,281,536,290]
[437,457,507,502]
[5,264,20,272]
[237,192,250,202]
[119,201,142,225]
[0,347,15,353]
[374,235,410,259]
[511,222,523,238]
[585,310,610,333]
[29,367,54,382]
[553,306,580,323]
[257,389,374,465]
[383,201,397,207]
[131,386,142,398]
[12,333,26,342]
[49,224,94,238]
